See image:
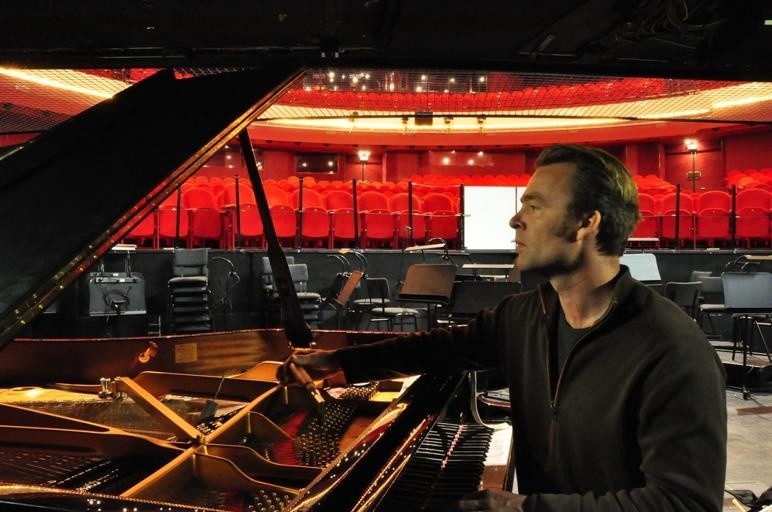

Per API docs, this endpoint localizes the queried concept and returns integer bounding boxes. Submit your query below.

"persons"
[273,140,732,512]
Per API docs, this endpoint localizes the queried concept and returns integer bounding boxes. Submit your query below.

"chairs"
[434,280,521,330]
[352,281,390,331]
[271,264,325,329]
[646,283,663,296]
[720,271,771,365]
[663,281,702,322]
[691,269,713,330]
[699,277,730,341]
[261,255,302,313]
[365,278,419,331]
[207,257,240,321]
[168,247,214,335]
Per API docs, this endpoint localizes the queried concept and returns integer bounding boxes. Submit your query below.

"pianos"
[1,55,514,512]
